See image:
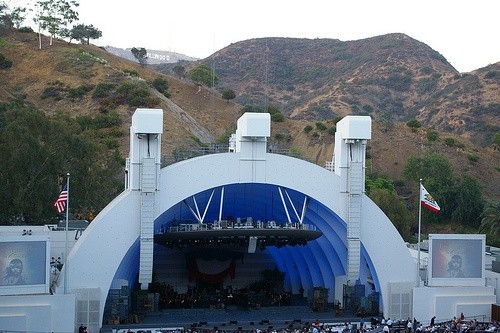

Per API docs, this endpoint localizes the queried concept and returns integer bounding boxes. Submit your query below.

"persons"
[78,285,500,333]
[4,258,33,285]
[447,255,465,278]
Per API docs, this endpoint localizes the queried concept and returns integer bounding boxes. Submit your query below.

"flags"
[420,183,441,213]
[54,182,68,214]
